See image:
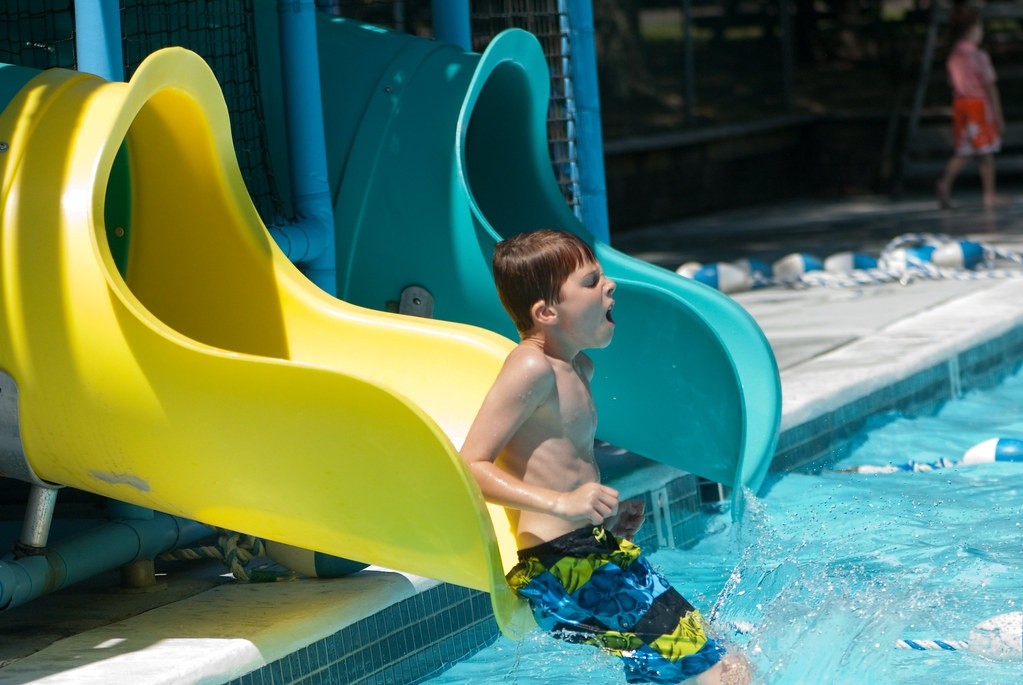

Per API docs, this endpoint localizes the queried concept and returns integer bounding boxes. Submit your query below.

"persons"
[460,230,753,685]
[936,16,1005,220]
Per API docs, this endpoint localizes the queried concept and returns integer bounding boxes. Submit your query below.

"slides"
[7,8,777,634]
